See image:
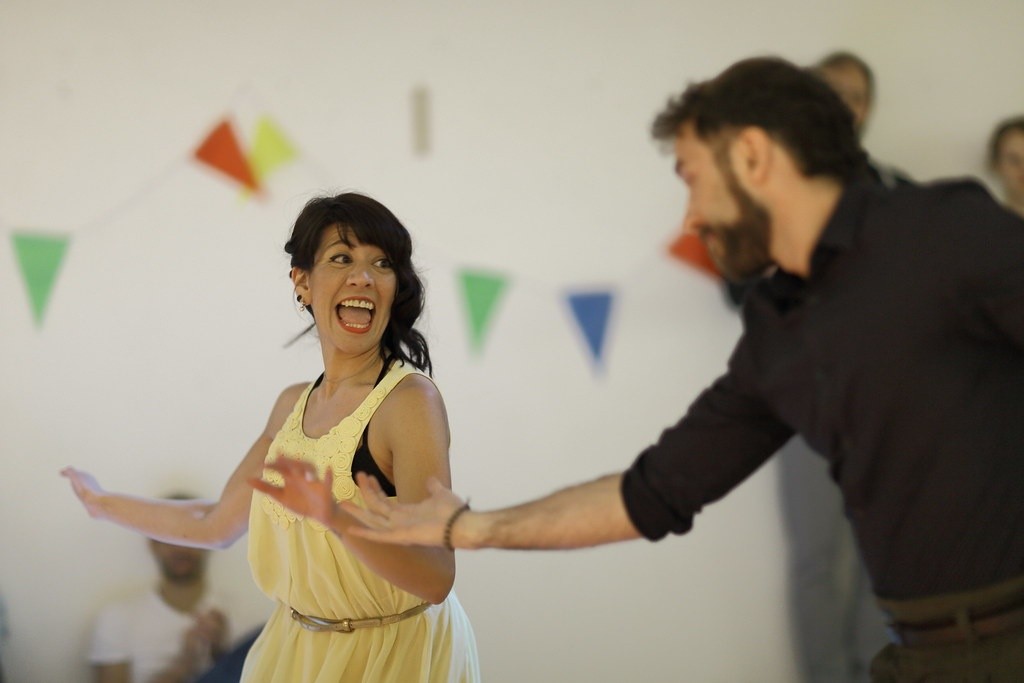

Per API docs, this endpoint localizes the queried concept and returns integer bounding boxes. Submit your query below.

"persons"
[59,194,481,683]
[91,494,269,683]
[984,117,1024,212]
[725,52,914,683]
[341,58,1024,683]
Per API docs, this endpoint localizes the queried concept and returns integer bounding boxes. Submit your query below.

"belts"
[289,601,432,633]
[884,605,1024,647]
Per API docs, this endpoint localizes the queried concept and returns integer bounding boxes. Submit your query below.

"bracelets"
[444,505,470,551]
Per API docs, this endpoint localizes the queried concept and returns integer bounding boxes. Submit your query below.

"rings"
[305,473,317,481]
